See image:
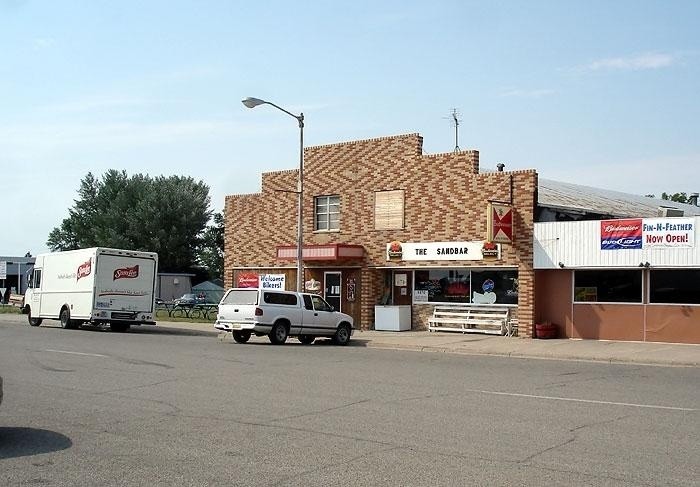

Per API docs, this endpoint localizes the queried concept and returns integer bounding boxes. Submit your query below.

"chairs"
[505,318,518,337]
[155,293,220,319]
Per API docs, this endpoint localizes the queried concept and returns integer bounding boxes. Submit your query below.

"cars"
[173,292,206,304]
[443,274,469,300]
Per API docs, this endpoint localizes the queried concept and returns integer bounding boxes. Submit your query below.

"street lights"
[239,95,306,291]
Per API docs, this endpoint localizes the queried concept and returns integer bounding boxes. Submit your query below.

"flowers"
[390,240,400,251]
[481,242,498,250]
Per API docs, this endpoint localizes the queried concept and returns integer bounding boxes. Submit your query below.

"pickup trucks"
[212,285,356,346]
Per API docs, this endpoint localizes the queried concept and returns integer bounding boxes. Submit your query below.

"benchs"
[428,303,510,337]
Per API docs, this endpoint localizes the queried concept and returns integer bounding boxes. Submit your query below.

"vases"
[389,251,402,258]
[484,250,498,257]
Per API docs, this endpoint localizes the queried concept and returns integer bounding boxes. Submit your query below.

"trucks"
[17,245,161,330]
